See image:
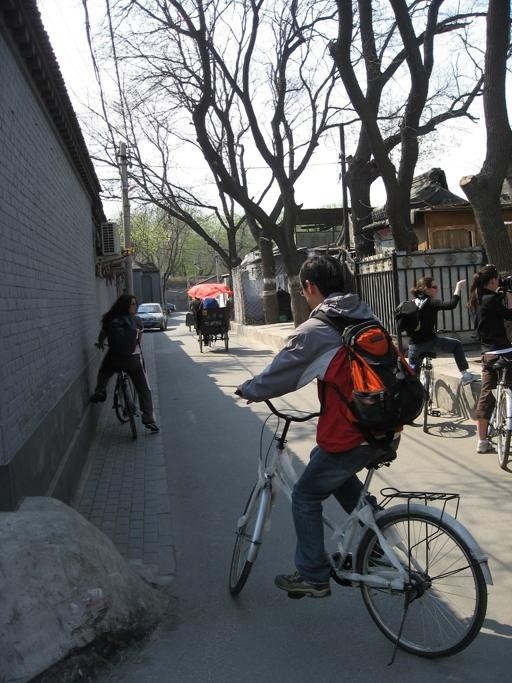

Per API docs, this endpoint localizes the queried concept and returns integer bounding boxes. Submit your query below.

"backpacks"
[310,309,431,450]
[104,310,137,356]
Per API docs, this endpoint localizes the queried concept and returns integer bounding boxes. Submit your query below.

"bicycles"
[95,342,146,440]
[403,328,443,433]
[470,330,511,469]
[225,386,493,670]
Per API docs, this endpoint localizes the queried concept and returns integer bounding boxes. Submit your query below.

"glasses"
[299,282,314,297]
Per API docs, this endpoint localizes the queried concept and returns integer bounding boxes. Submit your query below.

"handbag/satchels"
[393,301,425,340]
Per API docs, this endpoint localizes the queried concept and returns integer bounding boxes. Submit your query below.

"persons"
[198,295,219,310]
[465,262,512,454]
[234,252,426,598]
[88,293,160,431]
[189,296,203,329]
[392,275,480,423]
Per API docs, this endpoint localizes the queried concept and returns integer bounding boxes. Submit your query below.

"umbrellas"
[187,282,233,300]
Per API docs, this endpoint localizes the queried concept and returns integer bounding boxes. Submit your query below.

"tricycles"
[184,312,194,334]
[187,282,235,353]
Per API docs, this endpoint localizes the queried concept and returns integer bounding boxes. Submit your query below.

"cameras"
[499,274,512,290]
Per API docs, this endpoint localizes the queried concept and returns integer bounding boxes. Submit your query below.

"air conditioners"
[100,222,121,256]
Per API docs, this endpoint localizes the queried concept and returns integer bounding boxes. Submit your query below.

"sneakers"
[89,389,107,403]
[273,570,331,598]
[461,373,481,386]
[477,441,496,453]
[145,422,160,432]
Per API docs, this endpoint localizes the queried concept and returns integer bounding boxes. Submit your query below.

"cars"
[167,302,175,313]
[135,302,169,331]
